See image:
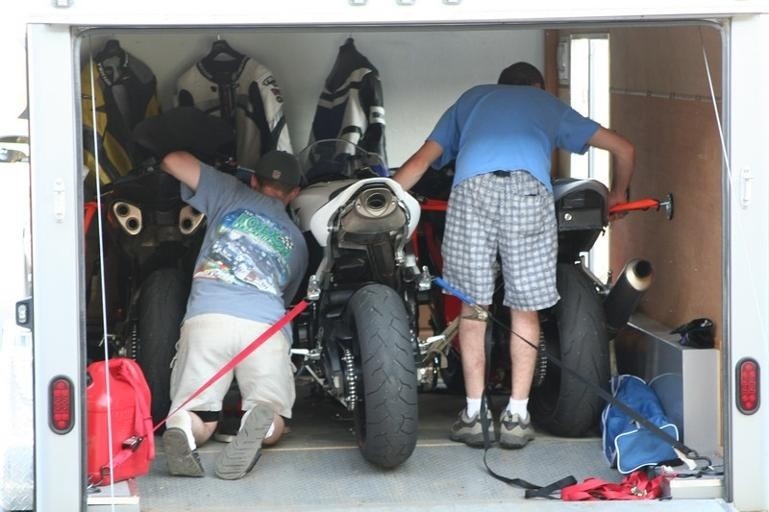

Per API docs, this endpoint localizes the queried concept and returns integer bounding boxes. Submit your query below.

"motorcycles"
[412,168,653,438]
[246,135,445,470]
[90,104,243,435]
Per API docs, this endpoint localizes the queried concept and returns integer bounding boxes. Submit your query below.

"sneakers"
[499,409,534,447]
[449,407,495,444]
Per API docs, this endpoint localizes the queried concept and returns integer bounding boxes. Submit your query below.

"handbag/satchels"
[601,375,679,473]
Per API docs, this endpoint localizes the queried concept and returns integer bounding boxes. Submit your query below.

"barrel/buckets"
[87,357,157,487]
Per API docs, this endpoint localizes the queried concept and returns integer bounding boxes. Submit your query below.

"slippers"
[162,428,204,477]
[212,403,275,480]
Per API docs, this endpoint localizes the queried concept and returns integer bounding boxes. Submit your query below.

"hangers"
[341,32,359,48]
[99,40,125,62]
[200,31,243,70]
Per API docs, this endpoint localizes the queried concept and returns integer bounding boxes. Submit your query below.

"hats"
[256,150,300,190]
[669,318,714,346]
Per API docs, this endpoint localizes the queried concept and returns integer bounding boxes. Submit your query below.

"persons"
[386,60,639,452]
[159,146,313,479]
